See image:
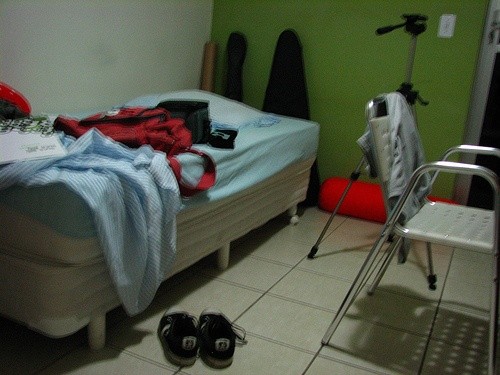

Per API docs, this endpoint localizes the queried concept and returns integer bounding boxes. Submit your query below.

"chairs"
[319,91,500,375]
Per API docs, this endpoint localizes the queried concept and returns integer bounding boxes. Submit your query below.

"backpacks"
[53,107,217,197]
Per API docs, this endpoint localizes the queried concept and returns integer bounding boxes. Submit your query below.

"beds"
[0,108,319,352]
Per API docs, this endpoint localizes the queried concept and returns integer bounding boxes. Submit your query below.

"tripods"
[307,12,437,292]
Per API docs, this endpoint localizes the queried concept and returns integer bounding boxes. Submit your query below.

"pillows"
[110,89,282,128]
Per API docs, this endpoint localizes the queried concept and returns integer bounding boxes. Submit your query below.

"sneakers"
[156,309,197,367]
[199,304,236,368]
